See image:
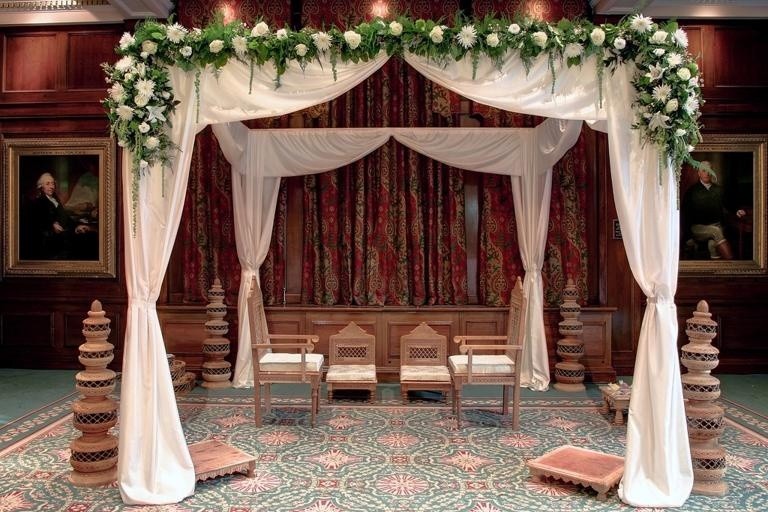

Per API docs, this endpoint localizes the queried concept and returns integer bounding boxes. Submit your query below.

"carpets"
[0,373,766,512]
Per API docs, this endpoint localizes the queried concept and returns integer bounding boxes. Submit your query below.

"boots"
[710,256,721,259]
[717,238,734,261]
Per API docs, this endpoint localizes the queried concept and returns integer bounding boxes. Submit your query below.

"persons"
[683,160,747,260]
[32,172,90,260]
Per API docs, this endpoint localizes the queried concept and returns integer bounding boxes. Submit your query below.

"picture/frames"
[679,132,768,277]
[0,137,117,280]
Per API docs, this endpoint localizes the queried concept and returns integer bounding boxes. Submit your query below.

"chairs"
[246,274,324,428]
[399,321,452,404]
[326,320,379,402]
[447,276,528,430]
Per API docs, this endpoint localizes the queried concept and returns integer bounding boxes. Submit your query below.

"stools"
[187,438,258,483]
[524,445,628,500]
